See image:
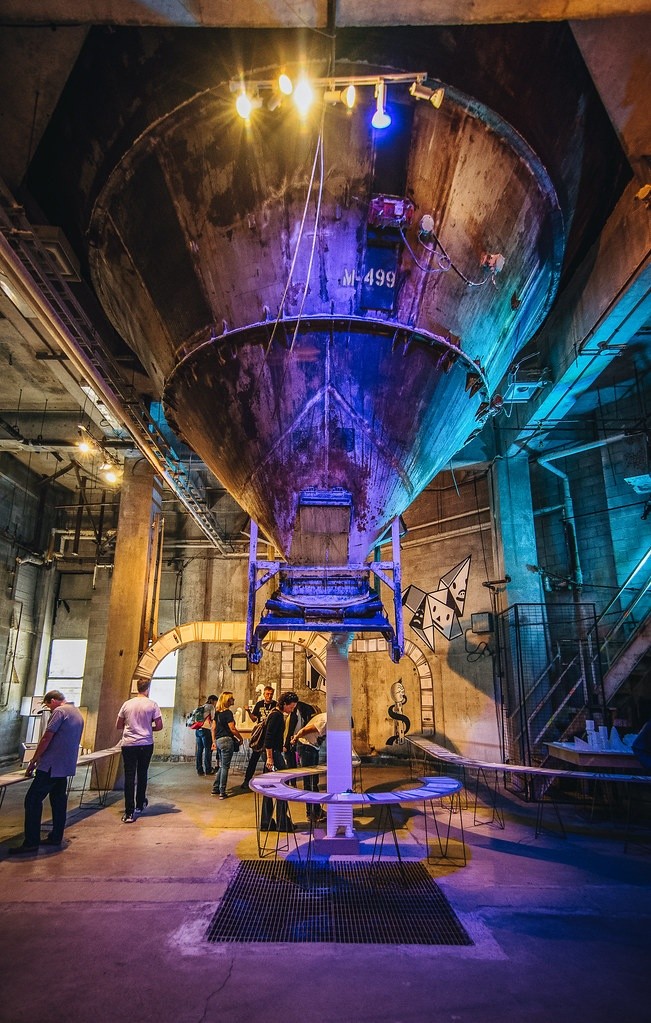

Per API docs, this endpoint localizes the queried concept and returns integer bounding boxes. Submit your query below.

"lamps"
[407,76,446,109]
[321,84,357,109]
[236,90,263,118]
[267,74,294,113]
[370,82,392,128]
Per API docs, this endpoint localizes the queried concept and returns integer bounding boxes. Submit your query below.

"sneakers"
[135,798,148,814]
[122,814,134,823]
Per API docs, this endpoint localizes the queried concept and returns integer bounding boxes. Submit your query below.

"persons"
[116,679,163,823]
[11,689,84,852]
[211,691,244,800]
[259,692,300,832]
[239,686,280,794]
[290,712,355,823]
[213,748,221,772]
[282,706,299,790]
[194,695,216,775]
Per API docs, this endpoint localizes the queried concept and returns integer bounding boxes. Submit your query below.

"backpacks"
[187,705,211,729]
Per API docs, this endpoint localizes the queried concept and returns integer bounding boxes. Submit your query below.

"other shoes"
[261,824,297,832]
[211,792,219,795]
[241,782,249,788]
[219,794,227,800]
[307,811,327,822]
[198,772,205,776]
[208,769,217,775]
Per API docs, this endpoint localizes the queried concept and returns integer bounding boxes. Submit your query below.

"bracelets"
[30,759,34,763]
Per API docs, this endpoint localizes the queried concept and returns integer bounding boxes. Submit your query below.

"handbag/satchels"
[248,711,284,752]
[233,737,240,752]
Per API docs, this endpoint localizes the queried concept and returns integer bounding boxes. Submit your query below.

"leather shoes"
[40,837,61,845]
[9,845,39,853]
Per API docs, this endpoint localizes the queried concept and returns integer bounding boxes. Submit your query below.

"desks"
[405,733,650,846]
[249,763,467,889]
[0,747,126,814]
